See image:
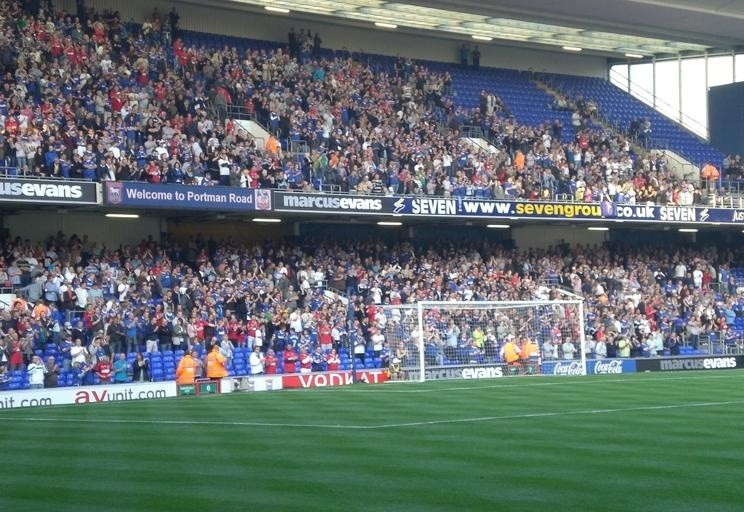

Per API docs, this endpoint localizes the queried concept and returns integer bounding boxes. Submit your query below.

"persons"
[1,235,743,393]
[2,1,744,211]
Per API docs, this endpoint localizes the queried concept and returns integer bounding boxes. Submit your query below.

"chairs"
[318,48,395,73]
[664,267,744,355]
[0,343,251,390]
[276,348,383,374]
[182,29,288,59]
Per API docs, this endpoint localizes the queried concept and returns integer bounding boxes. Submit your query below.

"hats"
[393,358,401,363]
